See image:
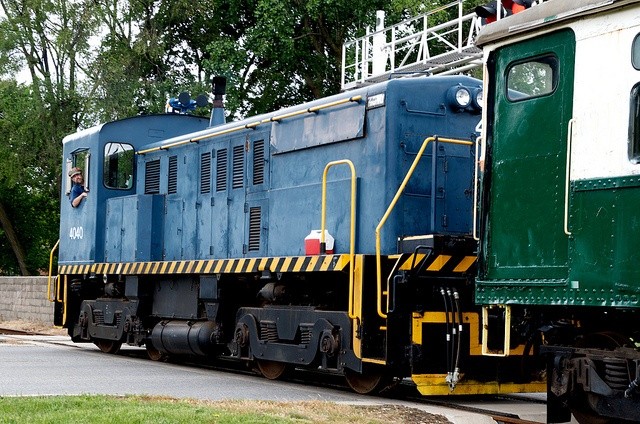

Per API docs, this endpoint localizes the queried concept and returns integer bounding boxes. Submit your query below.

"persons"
[68,166,87,207]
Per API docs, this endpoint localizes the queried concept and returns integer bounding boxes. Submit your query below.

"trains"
[48,0,640,423]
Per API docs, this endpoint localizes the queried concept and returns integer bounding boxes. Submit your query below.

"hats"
[68,168,82,178]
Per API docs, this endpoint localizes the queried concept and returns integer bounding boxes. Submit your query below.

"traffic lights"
[500,0,531,14]
[474,5,503,24]
